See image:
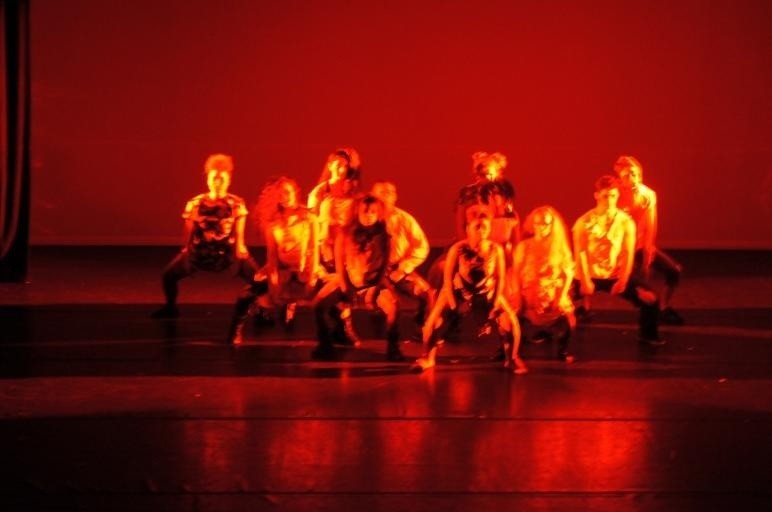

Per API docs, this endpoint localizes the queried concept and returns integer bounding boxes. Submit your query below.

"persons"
[149,146,680,376]
